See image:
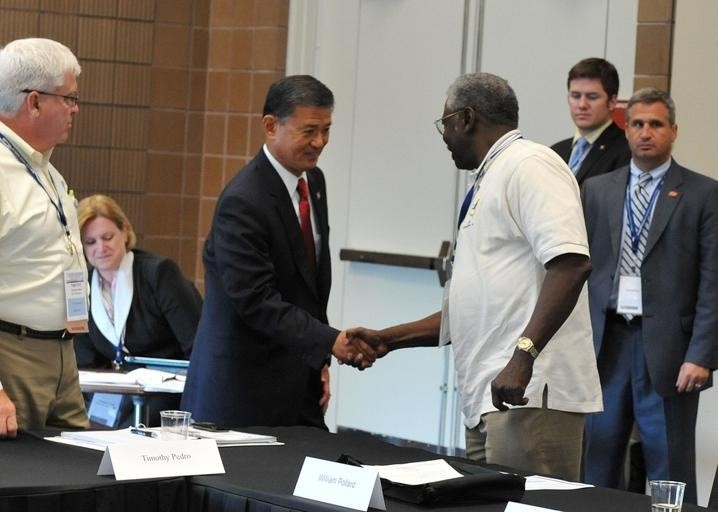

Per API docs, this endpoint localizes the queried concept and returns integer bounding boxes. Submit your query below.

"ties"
[571,138,587,170]
[296,178,316,267]
[619,171,652,321]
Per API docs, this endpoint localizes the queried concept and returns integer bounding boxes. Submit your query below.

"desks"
[74,364,188,428]
[1,425,716,512]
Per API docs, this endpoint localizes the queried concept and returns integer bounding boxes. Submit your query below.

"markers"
[131,429,158,438]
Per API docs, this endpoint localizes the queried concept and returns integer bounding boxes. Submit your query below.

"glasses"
[22,89,78,106]
[435,110,465,136]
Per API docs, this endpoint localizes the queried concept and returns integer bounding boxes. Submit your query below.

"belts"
[1,321,74,341]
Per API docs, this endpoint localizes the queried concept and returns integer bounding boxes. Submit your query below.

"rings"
[694,383,702,388]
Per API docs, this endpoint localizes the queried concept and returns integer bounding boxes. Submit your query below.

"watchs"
[517,335,539,358]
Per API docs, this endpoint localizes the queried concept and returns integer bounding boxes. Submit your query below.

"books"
[74,358,185,395]
[44,420,283,456]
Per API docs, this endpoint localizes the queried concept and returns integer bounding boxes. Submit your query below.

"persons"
[575,89,717,511]
[330,73,602,485]
[0,34,100,433]
[539,55,632,184]
[184,77,374,430]
[72,186,202,374]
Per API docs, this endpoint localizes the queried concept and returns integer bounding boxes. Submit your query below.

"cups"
[158,410,191,443]
[649,479,687,512]
